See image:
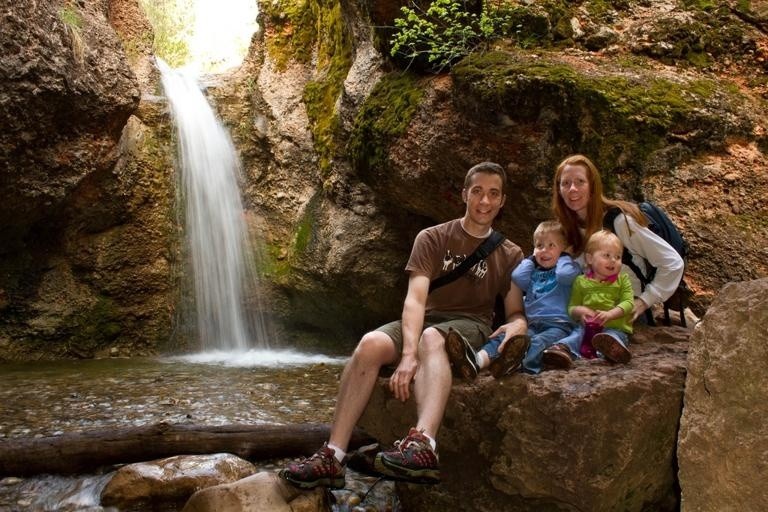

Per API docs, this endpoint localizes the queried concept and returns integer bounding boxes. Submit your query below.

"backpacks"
[602,201,685,289]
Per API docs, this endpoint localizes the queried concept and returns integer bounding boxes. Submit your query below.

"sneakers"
[445,329,480,380]
[278,442,349,489]
[374,427,442,484]
[489,335,531,378]
[591,332,632,364]
[542,343,572,368]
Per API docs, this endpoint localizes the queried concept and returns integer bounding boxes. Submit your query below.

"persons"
[550,153,686,337]
[276,160,526,492]
[440,219,586,383]
[541,227,638,371]
